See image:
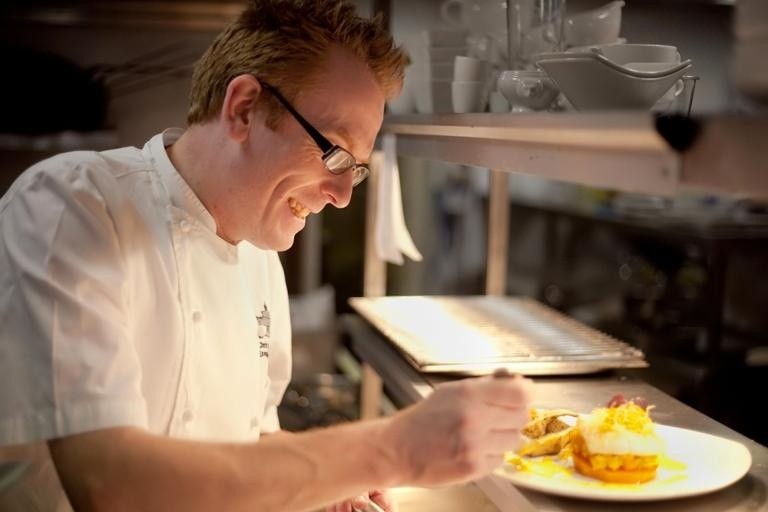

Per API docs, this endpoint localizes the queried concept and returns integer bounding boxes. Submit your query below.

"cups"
[449,55,493,115]
[497,69,558,114]
[506,0,568,79]
[608,43,685,114]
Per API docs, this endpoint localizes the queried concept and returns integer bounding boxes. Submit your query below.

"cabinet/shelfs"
[344,94,765,511]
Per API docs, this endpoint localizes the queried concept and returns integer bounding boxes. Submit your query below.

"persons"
[1,0,532,511]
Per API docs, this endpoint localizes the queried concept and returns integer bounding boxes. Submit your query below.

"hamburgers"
[570,403,659,484]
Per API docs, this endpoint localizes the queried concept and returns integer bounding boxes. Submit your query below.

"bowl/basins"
[406,30,472,116]
[533,51,692,115]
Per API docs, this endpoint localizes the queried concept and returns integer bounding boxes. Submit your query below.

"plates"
[490,414,754,503]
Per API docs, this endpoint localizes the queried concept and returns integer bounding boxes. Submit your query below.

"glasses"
[258,80,372,189]
